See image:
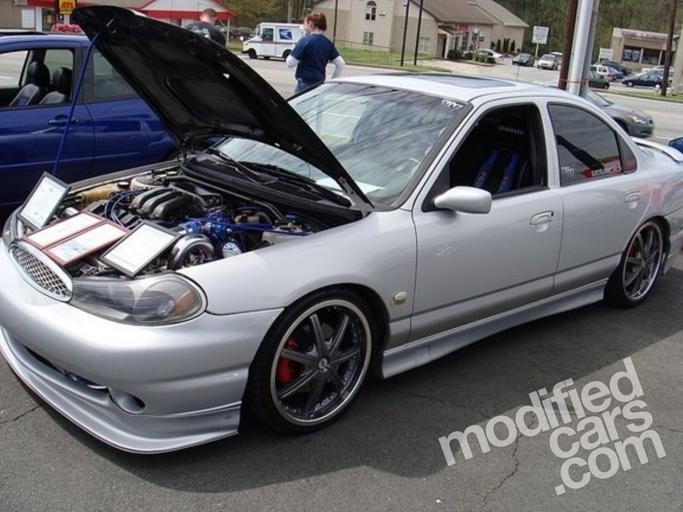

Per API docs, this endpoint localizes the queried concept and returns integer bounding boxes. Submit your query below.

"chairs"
[466,112,543,193]
[6,62,71,105]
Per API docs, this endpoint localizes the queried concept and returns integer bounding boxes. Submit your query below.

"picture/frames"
[12,171,182,277]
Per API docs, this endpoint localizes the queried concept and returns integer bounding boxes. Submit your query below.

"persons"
[182,8,226,49]
[285,12,345,95]
